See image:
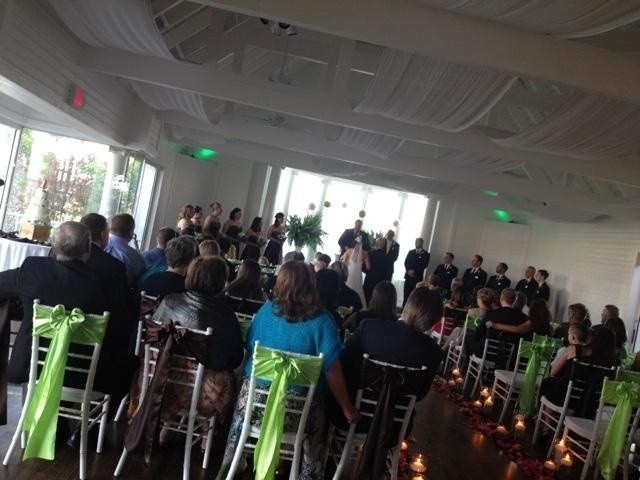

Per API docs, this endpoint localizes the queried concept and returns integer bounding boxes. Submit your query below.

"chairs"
[557,376,639,480]
[226,340,324,480]
[443,316,485,379]
[462,335,515,399]
[614,364,634,383]
[531,331,564,348]
[0,298,111,480]
[532,356,604,459]
[234,311,256,327]
[114,312,218,480]
[491,336,558,424]
[337,353,427,479]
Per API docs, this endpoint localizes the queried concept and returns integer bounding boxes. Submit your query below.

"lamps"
[485,396,494,406]
[562,453,572,466]
[474,400,482,407]
[398,440,408,468]
[449,379,455,386]
[453,367,460,377]
[544,457,556,472]
[410,452,428,480]
[456,377,463,383]
[480,387,489,396]
[64,82,86,110]
[555,438,567,453]
[515,419,526,431]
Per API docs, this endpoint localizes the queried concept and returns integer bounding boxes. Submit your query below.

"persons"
[532,269,551,302]
[139,226,179,270]
[460,286,533,398]
[512,266,538,297]
[342,234,371,312]
[486,299,554,344]
[263,251,306,301]
[241,217,267,261]
[48,212,127,291]
[401,238,430,308]
[202,201,223,245]
[381,230,399,263]
[540,326,619,445]
[139,235,199,319]
[485,261,511,289]
[175,204,196,236]
[440,288,495,372]
[314,251,332,271]
[0,222,141,452]
[363,237,394,306]
[445,277,465,306]
[549,322,589,369]
[420,275,441,292]
[340,280,398,336]
[462,254,488,301]
[262,212,288,265]
[602,317,626,362]
[338,220,370,251]
[229,261,361,480]
[221,259,269,319]
[549,303,592,342]
[593,304,620,332]
[429,252,458,300]
[100,213,148,276]
[221,207,246,262]
[198,238,222,258]
[514,292,530,316]
[328,260,349,283]
[192,205,203,234]
[130,256,243,458]
[466,286,480,308]
[315,268,362,315]
[325,286,445,448]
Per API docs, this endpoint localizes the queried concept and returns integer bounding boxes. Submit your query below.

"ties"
[417,249,420,254]
[354,230,359,238]
[445,264,448,270]
[472,267,476,273]
[495,275,500,281]
